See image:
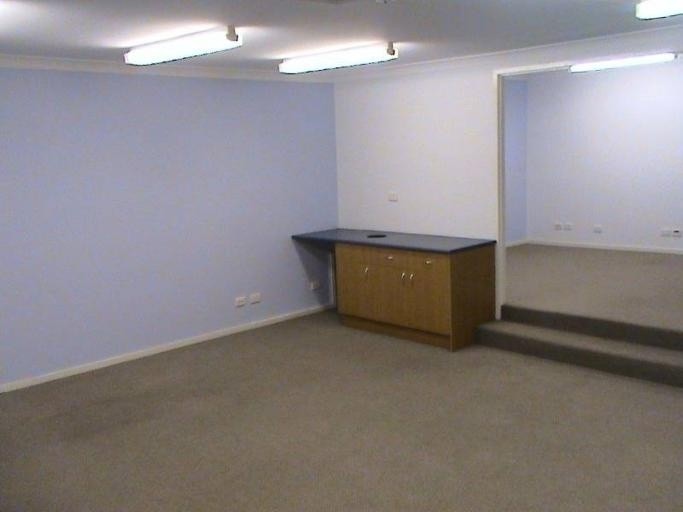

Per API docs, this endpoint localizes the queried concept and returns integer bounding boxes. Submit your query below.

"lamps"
[278,42,399,74]
[569,53,678,76]
[123,24,244,67]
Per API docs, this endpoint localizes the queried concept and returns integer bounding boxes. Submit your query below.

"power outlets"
[235,293,260,307]
[310,280,320,289]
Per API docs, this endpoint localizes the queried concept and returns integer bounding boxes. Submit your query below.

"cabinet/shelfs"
[292,228,497,352]
